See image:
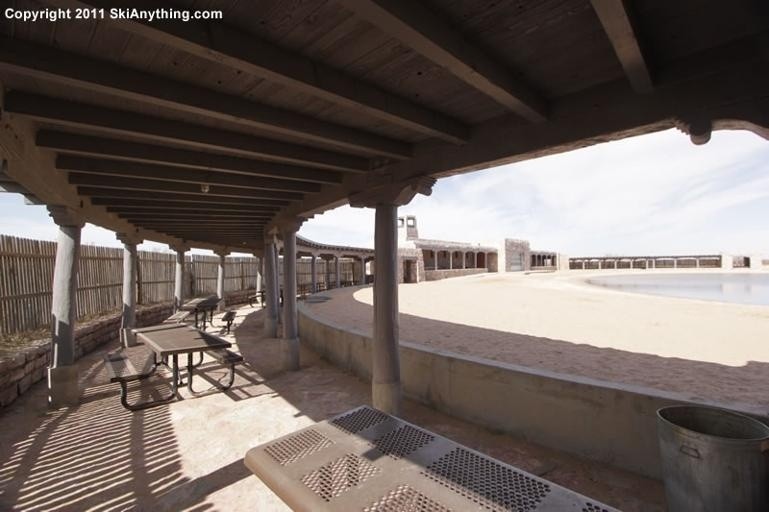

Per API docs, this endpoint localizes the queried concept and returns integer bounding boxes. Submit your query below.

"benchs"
[248,283,353,308]
[192,348,243,393]
[163,311,190,323]
[219,312,236,337]
[103,349,142,410]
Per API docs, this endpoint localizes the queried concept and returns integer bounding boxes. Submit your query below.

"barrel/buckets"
[657,405,768,512]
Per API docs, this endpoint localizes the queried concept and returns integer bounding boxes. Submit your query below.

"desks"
[131,322,229,413]
[181,298,221,335]
[244,404,622,511]
